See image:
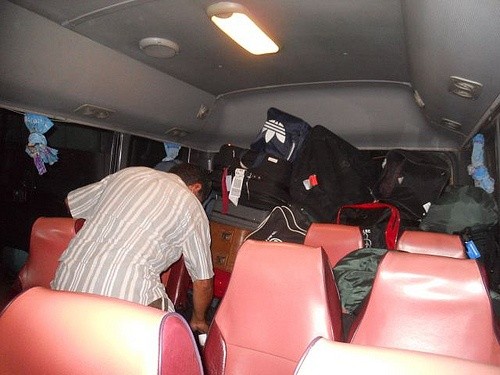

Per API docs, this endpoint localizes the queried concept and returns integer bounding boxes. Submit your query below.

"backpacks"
[367,149,459,226]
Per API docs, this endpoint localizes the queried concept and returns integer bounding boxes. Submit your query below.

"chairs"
[201,239,342,375]
[292,335,500,375]
[396,230,467,260]
[0,216,85,312]
[303,224,364,267]
[0,285,204,375]
[347,248,500,366]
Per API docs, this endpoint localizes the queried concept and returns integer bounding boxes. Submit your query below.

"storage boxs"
[206,198,270,272]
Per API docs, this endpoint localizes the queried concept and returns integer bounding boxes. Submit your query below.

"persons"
[50,163,216,333]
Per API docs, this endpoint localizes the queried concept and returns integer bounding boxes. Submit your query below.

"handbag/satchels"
[250,107,312,164]
[238,153,293,213]
[288,124,384,226]
[207,144,238,190]
[226,158,263,201]
[244,195,312,244]
[329,228,388,316]
[452,223,500,295]
[421,185,500,234]
